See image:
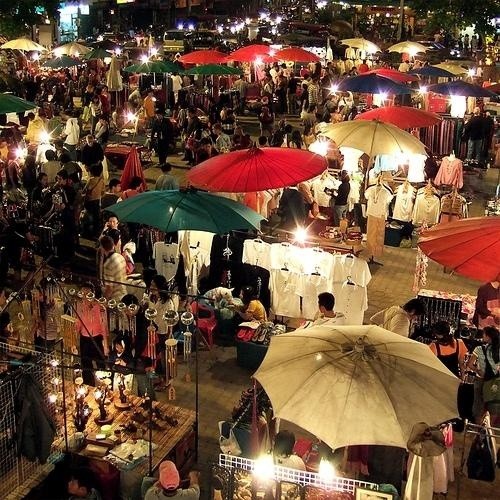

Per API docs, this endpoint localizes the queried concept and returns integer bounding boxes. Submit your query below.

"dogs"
[202,287,235,310]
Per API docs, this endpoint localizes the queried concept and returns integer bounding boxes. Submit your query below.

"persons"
[0,1,499,500]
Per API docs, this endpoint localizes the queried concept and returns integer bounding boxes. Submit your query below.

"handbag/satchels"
[482,361,499,404]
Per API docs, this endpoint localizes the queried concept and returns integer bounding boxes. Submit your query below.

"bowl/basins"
[318,232,343,243]
[342,237,363,245]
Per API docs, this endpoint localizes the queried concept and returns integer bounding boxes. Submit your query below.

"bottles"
[114,430,121,443]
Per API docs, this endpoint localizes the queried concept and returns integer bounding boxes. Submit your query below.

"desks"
[103,142,148,164]
[49,377,200,497]
[416,288,478,320]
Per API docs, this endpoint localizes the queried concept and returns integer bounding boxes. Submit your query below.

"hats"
[159,460,180,491]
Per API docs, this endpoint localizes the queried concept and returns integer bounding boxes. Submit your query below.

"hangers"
[319,169,459,202]
[232,388,270,431]
[164,236,362,288]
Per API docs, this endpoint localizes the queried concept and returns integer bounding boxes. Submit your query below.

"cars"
[417,41,460,58]
[96,0,330,54]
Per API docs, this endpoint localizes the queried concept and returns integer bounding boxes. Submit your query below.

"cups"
[100,425,112,437]
